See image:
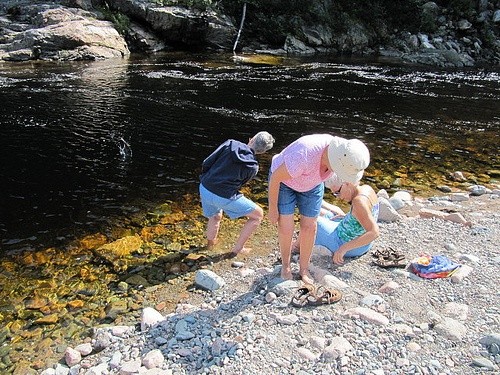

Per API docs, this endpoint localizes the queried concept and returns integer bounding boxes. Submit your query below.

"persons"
[199,131,275,255]
[268,133,380,285]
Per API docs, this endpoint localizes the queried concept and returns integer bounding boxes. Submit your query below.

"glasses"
[332,185,343,197]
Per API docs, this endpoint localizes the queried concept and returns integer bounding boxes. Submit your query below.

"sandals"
[291,283,342,307]
[372,245,408,268]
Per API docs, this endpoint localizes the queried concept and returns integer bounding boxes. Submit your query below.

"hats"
[328,135,371,183]
